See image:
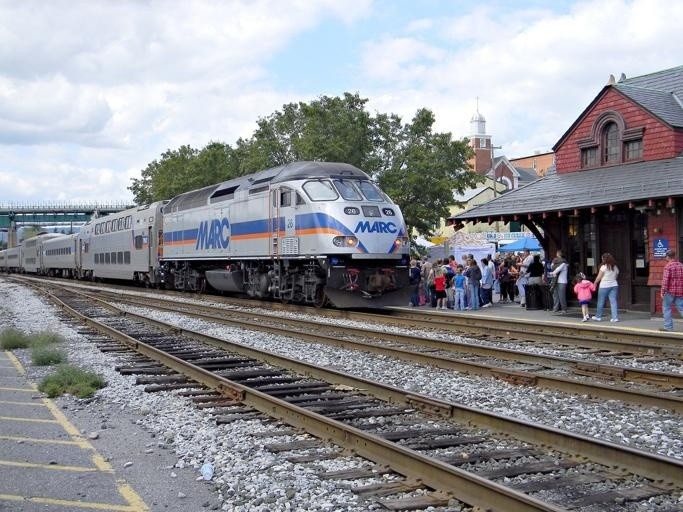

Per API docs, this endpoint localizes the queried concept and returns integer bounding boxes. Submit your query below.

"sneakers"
[592,316,602,321]
[660,327,673,332]
[610,319,619,323]
[582,317,587,321]
[412,298,526,310]
[586,314,589,320]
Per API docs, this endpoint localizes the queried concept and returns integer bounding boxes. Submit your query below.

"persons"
[571,272,598,321]
[658,250,683,331]
[591,253,620,322]
[406,243,569,323]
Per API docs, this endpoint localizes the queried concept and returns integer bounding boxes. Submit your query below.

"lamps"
[567,215,580,240]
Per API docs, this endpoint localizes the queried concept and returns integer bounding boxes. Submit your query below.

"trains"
[0,157,426,312]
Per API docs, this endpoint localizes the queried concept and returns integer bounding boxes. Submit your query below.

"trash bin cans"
[523,284,553,310]
